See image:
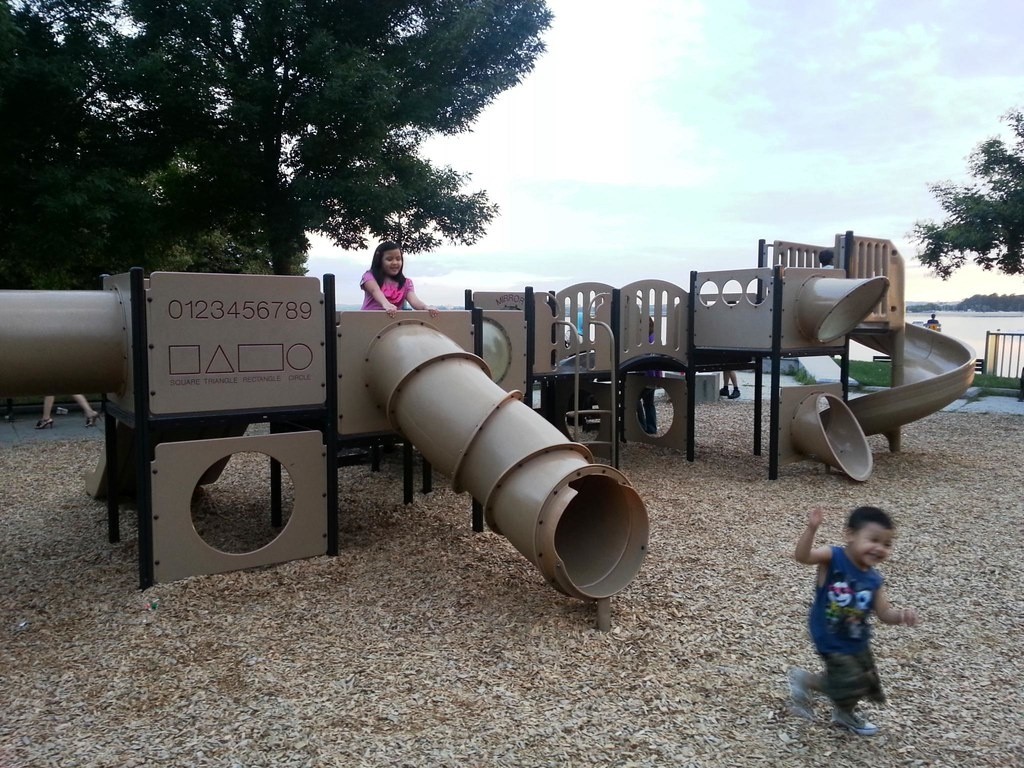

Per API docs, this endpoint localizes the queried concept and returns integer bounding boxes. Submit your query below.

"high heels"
[85,411,102,427]
[36,419,54,429]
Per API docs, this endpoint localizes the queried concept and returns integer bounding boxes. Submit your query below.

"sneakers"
[834,705,876,736]
[788,668,815,718]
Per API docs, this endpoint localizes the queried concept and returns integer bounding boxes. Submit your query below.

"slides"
[362,317,650,602]
[778,320,978,483]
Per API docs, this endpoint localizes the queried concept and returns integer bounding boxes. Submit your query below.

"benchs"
[665,371,720,404]
[872,355,984,374]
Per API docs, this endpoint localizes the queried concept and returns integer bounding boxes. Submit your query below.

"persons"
[720,371,740,399]
[360,241,439,318]
[638,316,663,434]
[789,505,915,735]
[33,394,102,429]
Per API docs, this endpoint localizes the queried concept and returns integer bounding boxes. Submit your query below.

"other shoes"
[719,388,729,395]
[729,390,740,399]
